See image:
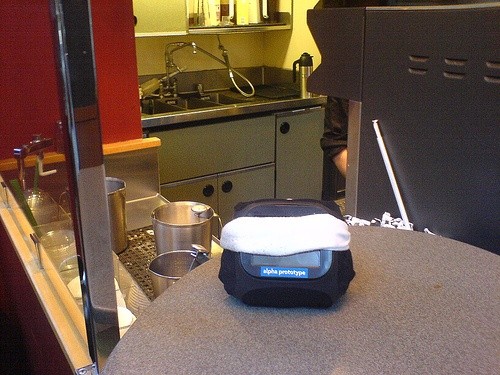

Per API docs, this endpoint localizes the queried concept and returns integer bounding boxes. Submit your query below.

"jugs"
[103,176,128,255]
[148,250,210,299]
[292,52,314,98]
[149,201,223,261]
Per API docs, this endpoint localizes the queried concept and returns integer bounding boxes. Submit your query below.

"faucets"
[13,134,58,192]
[196,83,211,100]
[159,41,197,100]
[138,84,143,100]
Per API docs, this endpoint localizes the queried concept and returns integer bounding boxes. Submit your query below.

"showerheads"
[164,42,255,97]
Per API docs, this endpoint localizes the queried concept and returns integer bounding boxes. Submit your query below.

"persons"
[320,95,348,200]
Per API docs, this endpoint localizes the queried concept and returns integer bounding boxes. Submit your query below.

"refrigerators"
[306,2,500,257]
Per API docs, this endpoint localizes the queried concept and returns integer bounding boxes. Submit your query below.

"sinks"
[140,99,187,118]
[194,93,252,105]
[163,97,223,110]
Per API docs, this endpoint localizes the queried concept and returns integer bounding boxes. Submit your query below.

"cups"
[18,184,82,310]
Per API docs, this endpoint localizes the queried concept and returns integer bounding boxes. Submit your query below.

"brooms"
[372,120,429,230]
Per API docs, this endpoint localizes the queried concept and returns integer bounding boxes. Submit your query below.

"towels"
[220,214,351,256]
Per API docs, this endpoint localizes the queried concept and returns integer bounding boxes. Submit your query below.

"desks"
[100,225,500,375]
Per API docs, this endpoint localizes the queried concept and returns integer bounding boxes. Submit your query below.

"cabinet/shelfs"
[141,103,326,239]
